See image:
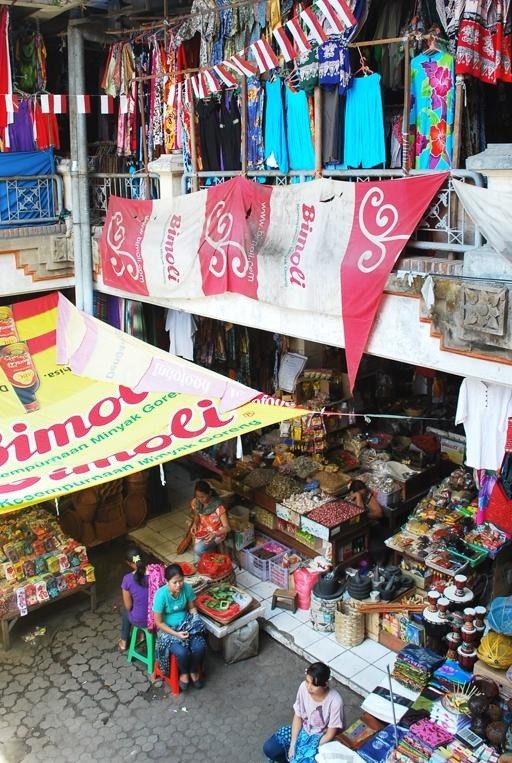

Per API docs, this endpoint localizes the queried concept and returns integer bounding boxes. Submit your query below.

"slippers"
[117,639,126,652]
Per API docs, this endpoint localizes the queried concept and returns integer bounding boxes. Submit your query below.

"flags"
[166,0,358,107]
[120,94,134,114]
[4,94,18,112]
[76,94,92,113]
[54,94,68,114]
[40,93,54,113]
[100,94,114,114]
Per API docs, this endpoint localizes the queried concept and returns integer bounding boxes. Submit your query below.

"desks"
[0,580,97,651]
[230,477,429,571]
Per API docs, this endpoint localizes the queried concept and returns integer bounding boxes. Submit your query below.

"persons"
[118,549,166,652]
[190,480,232,566]
[152,563,207,690]
[263,661,344,762]
[345,479,384,546]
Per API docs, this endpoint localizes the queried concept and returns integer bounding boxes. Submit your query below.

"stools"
[126,625,182,696]
[271,589,298,614]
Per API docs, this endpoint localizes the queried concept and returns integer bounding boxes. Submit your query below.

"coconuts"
[468,696,512,763]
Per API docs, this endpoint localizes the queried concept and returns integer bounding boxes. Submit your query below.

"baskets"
[61,488,149,543]
[206,566,237,587]
[335,601,365,647]
[233,528,310,590]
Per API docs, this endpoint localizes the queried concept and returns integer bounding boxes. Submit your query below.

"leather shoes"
[193,675,203,689]
[179,679,189,691]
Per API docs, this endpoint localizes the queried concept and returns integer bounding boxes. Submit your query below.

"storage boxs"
[223,500,310,590]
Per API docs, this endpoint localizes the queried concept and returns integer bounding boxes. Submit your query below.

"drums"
[421,574,486,672]
